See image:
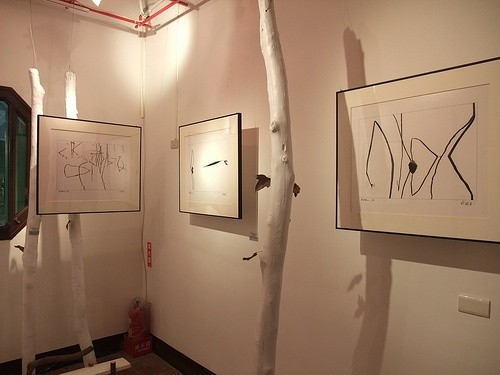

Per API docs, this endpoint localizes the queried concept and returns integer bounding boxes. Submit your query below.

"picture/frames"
[36,115,142,216]
[335,57,500,244]
[178,113,242,220]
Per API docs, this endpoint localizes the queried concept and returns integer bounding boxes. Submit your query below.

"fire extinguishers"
[128,299,147,341]
